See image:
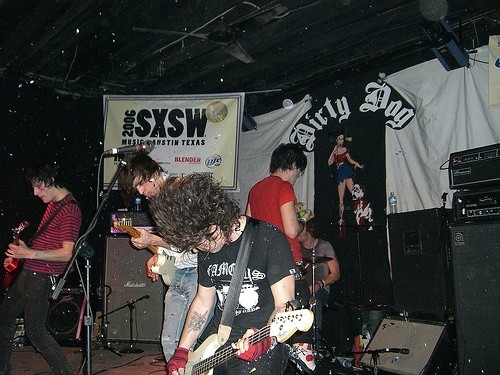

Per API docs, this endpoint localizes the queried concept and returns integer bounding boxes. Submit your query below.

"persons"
[245,142,312,266]
[298,221,342,295]
[148,172,298,375]
[118,155,218,363]
[0,153,83,375]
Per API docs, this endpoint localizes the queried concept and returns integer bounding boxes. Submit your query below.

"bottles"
[389,191,398,214]
[134,193,143,212]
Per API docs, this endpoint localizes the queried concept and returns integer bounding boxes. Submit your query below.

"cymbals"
[301,256,334,265]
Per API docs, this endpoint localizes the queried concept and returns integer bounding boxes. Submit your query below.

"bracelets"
[315,279,325,289]
[298,217,306,227]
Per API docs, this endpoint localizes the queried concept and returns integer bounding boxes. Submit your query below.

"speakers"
[24,290,87,348]
[450,224,500,375]
[388,209,458,375]
[102,235,168,344]
[358,315,457,375]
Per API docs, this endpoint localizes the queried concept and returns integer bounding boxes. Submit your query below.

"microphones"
[105,140,154,155]
[383,348,410,354]
[136,295,149,301]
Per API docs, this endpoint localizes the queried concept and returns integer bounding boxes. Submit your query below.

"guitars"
[168,310,312,375]
[2,219,29,289]
[112,219,178,285]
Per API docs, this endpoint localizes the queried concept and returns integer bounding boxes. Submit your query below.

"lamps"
[208,21,237,45]
